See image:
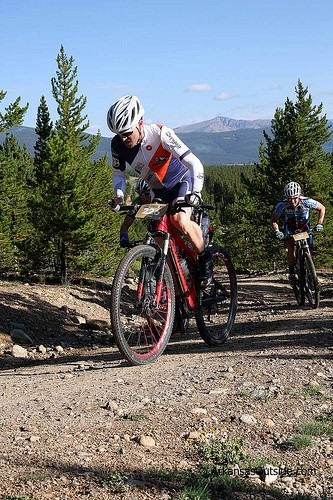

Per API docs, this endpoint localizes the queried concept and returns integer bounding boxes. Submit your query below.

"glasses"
[118,129,134,138]
[137,192,150,196]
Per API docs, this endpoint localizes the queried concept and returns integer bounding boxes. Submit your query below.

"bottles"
[201,214,211,245]
[180,257,193,288]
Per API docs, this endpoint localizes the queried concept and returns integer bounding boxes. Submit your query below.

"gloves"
[274,231,283,240]
[314,224,323,232]
[119,234,129,247]
[185,194,203,208]
[108,195,124,212]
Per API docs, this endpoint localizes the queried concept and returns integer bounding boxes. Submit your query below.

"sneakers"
[199,251,212,286]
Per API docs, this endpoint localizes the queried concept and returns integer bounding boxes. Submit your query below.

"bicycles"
[272,226,320,310]
[107,193,238,366]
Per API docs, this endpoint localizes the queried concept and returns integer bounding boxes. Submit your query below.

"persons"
[270,182,325,290]
[107,95,214,331]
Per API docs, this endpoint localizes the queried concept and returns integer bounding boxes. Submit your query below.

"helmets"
[107,95,144,134]
[136,177,151,192]
[284,182,301,198]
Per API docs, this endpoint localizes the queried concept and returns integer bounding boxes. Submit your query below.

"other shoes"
[308,278,315,291]
[288,275,296,288]
